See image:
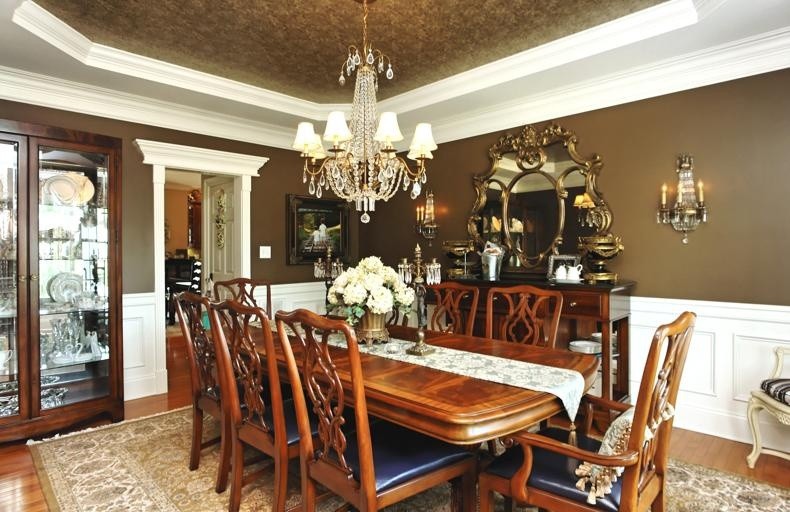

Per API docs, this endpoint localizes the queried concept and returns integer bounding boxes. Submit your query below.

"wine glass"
[49,311,86,361]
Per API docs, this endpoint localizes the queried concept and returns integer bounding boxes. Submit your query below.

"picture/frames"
[283,193,351,265]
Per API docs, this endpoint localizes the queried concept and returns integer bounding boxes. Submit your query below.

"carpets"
[21,404,790,512]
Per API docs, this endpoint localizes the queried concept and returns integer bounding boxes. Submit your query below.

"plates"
[50,273,84,302]
[45,174,95,203]
[568,341,602,355]
[550,278,585,284]
[589,331,617,345]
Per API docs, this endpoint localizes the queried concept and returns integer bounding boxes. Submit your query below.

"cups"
[0,350,13,369]
[481,255,503,281]
[555,265,567,279]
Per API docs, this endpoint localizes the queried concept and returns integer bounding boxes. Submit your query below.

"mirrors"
[464,122,614,279]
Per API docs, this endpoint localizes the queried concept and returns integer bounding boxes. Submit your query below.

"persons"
[319,217,328,248]
[309,226,320,250]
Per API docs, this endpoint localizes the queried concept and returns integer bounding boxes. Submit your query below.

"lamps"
[295,0,437,224]
[659,154,709,246]
[411,188,441,249]
[574,189,595,227]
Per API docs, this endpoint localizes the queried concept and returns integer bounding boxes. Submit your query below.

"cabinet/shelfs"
[429,279,639,408]
[1,120,125,443]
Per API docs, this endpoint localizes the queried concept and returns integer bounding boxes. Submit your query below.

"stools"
[744,346,790,471]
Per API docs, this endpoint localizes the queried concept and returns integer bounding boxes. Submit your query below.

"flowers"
[323,256,414,316]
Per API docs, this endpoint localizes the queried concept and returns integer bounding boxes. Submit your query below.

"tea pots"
[568,264,583,280]
